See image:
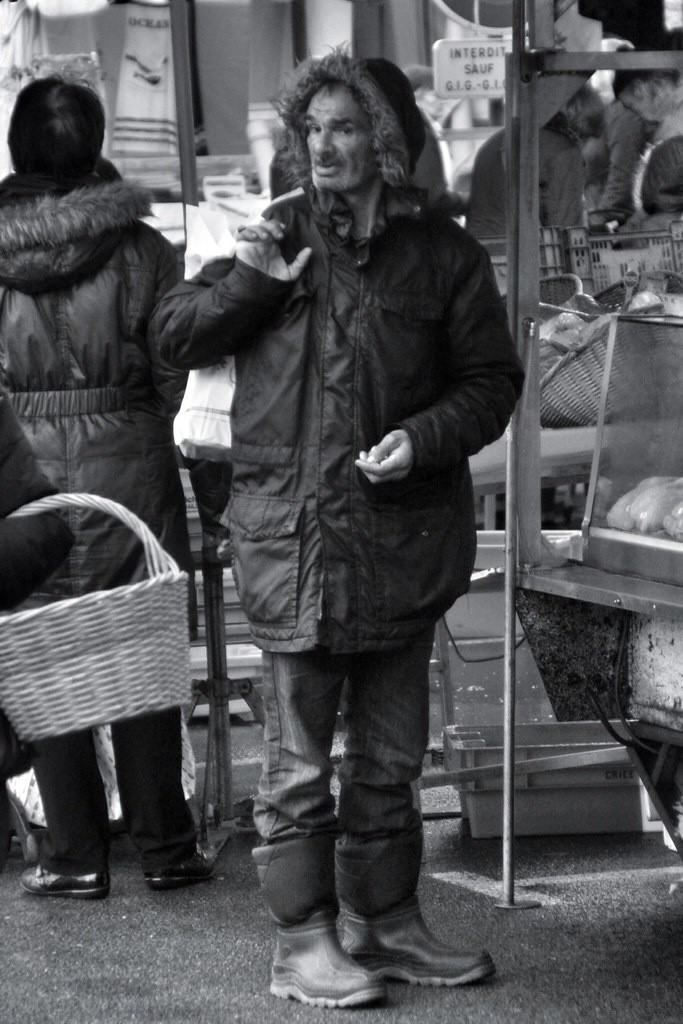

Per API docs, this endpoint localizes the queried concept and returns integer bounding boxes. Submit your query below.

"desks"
[181,428,616,876]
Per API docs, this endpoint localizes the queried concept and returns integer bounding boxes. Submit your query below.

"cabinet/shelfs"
[499,0,683,911]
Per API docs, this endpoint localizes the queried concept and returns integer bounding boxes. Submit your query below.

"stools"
[181,642,266,742]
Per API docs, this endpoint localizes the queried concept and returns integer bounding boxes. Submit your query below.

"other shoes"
[143,861,221,890]
[20,866,113,900]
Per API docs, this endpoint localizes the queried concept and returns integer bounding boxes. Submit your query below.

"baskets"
[0,493,194,742]
[535,274,600,337]
[538,275,660,420]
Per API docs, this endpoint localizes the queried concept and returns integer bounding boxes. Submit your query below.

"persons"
[411,27,683,238]
[0,387,76,780]
[151,42,524,1007]
[0,74,215,896]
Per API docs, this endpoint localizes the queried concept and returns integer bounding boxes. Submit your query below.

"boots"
[332,837,504,989]
[251,835,418,1008]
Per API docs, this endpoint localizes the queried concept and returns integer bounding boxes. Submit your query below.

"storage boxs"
[444,719,645,840]
